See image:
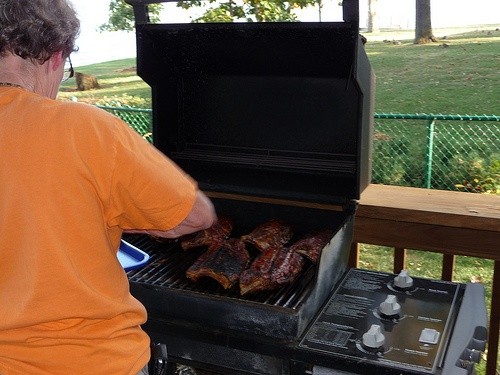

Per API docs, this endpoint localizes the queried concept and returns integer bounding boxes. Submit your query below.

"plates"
[116,239,149,272]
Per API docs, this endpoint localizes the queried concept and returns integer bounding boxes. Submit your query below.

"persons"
[0,0,216,375]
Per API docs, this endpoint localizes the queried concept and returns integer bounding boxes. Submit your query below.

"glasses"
[61,55,74,82]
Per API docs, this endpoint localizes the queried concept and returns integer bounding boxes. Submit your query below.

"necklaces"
[0,82,23,89]
